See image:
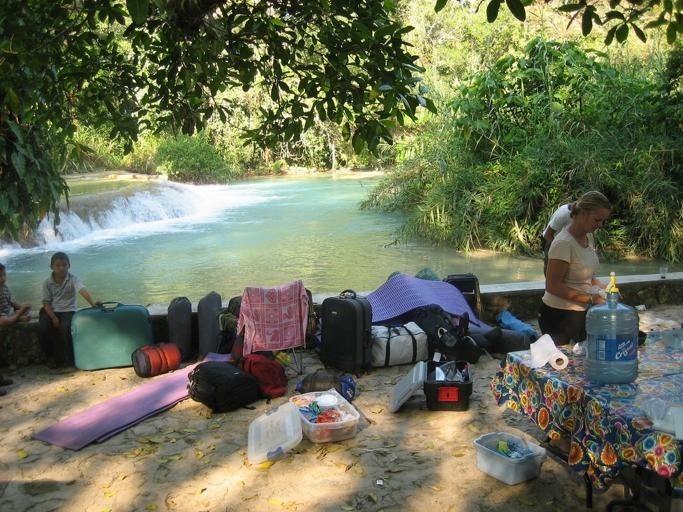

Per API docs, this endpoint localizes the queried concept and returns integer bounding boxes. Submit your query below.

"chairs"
[237,279,309,376]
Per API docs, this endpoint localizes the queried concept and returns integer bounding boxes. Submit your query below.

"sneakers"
[63,353,73,362]
[47,358,57,368]
[0,375,13,386]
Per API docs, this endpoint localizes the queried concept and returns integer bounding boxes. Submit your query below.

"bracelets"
[51,314,56,320]
[590,294,594,306]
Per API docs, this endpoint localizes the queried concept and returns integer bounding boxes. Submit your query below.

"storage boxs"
[389,359,474,413]
[246,390,362,465]
[473,430,547,486]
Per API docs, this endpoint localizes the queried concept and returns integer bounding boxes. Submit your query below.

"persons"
[541,203,575,277]
[536,191,611,346]
[39,252,97,369]
[0,304,32,396]
[0,263,21,363]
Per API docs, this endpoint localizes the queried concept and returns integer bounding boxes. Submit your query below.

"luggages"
[442,274,479,320]
[320,290,373,378]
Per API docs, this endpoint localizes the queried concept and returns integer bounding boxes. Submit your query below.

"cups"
[660,267,668,279]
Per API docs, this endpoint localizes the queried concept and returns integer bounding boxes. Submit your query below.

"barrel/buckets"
[585,293,639,383]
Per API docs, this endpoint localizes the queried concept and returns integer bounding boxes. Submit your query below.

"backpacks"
[186,354,288,413]
[414,309,503,364]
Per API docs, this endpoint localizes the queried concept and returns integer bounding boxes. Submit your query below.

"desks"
[507,328,683,511]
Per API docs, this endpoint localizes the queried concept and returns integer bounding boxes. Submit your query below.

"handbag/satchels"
[215,288,319,356]
[70,301,153,371]
[371,321,429,368]
